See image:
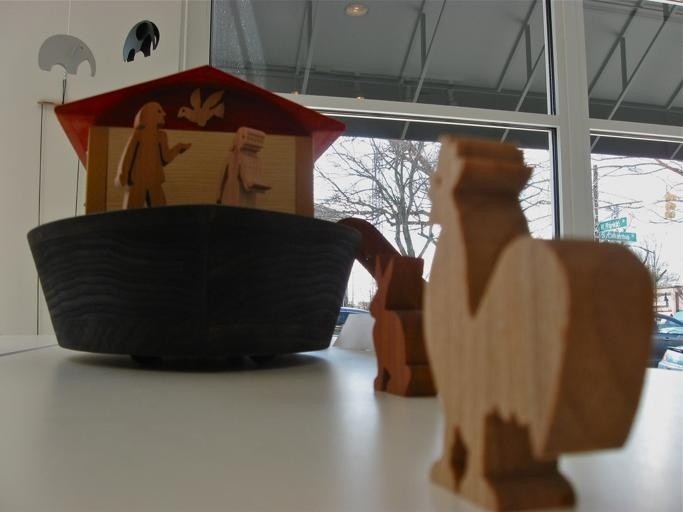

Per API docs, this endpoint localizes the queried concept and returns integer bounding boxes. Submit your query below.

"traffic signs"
[598,217,628,230]
[599,231,637,243]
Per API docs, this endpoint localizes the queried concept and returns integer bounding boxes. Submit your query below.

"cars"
[333,307,370,337]
[651,311,683,368]
